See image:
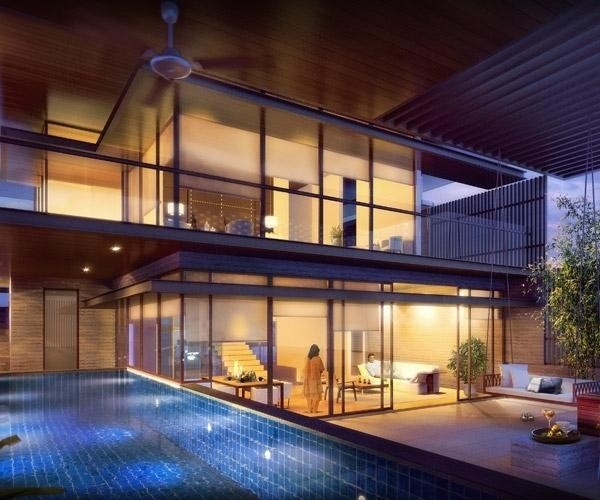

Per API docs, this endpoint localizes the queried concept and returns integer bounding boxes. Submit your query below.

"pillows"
[408,371,425,383]
[497,361,563,395]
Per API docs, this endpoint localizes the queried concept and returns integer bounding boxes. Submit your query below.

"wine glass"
[561,424,575,438]
[543,409,555,430]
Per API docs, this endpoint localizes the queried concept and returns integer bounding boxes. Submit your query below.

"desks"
[203,375,285,410]
[509,434,599,481]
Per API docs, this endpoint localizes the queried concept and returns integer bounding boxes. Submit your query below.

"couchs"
[357,361,441,395]
[483,372,599,407]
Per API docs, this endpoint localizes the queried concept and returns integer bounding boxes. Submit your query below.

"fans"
[105,2,276,109]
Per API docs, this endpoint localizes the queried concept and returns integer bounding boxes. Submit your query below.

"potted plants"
[328,225,343,247]
[446,334,490,394]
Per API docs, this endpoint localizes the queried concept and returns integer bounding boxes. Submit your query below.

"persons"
[303,343,325,413]
[366,353,413,380]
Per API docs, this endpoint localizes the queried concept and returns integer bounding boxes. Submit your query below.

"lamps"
[264,215,279,229]
[167,202,184,217]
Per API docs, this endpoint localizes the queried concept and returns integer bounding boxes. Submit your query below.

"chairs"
[225,218,254,236]
[323,368,357,403]
[221,385,243,397]
[251,380,293,408]
[381,234,414,254]
